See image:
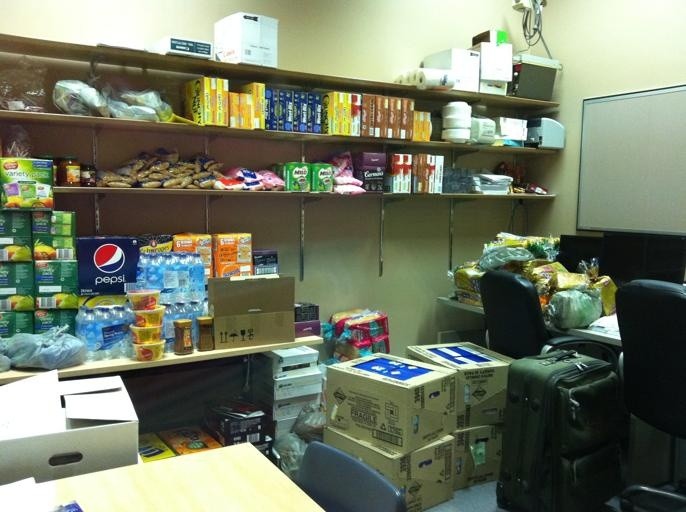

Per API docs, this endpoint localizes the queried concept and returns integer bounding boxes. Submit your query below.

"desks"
[0,332,322,433]
[433,290,622,349]
[31,442,327,512]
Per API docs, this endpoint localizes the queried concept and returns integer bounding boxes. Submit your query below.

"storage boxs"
[210,11,279,70]
[247,347,323,420]
[319,340,510,511]
[419,29,560,102]
[207,276,297,347]
[0,370,141,485]
[0,155,78,343]
[75,234,140,293]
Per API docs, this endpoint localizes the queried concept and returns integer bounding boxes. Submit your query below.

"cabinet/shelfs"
[52,154,323,207]
[0,31,562,203]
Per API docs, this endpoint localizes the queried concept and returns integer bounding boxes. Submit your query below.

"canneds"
[174,319,193,354]
[197,316,215,351]
[34,154,97,187]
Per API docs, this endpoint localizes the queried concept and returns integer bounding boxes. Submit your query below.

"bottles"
[76,302,201,361]
[136,251,206,301]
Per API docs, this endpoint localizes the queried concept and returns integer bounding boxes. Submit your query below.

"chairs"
[477,271,617,370]
[293,437,405,512]
[608,279,686,512]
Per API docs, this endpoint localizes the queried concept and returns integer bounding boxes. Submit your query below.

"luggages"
[496,344,631,512]
